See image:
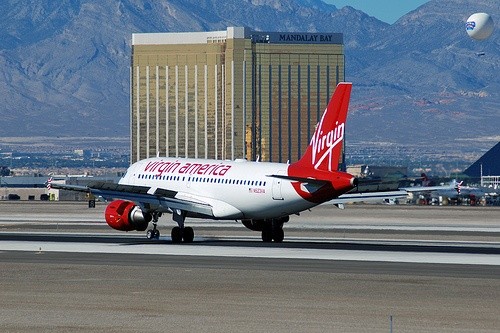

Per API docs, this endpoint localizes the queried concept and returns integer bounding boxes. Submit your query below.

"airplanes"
[44,81,424,244]
[398,178,500,207]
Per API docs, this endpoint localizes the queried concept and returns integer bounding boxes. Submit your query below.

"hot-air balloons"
[464,12,494,58]
